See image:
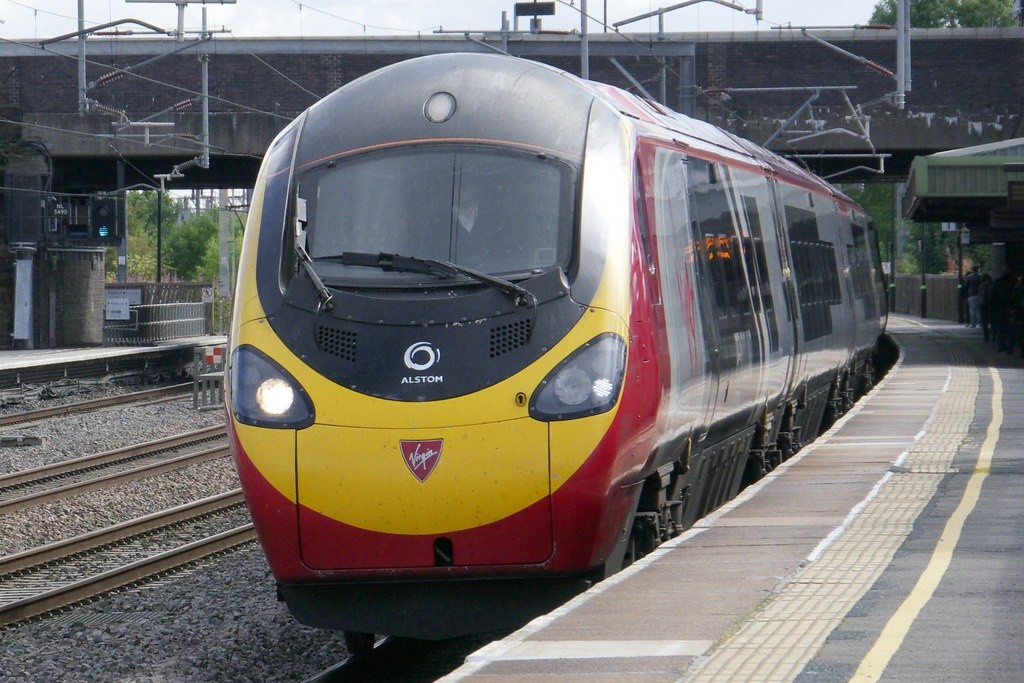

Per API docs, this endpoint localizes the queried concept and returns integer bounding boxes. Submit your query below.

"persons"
[964,266,1024,357]
[428,173,502,254]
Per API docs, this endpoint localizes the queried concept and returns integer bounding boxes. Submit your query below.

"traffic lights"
[92,197,117,242]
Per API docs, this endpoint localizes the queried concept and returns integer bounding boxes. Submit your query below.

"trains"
[223,52,888,656]
